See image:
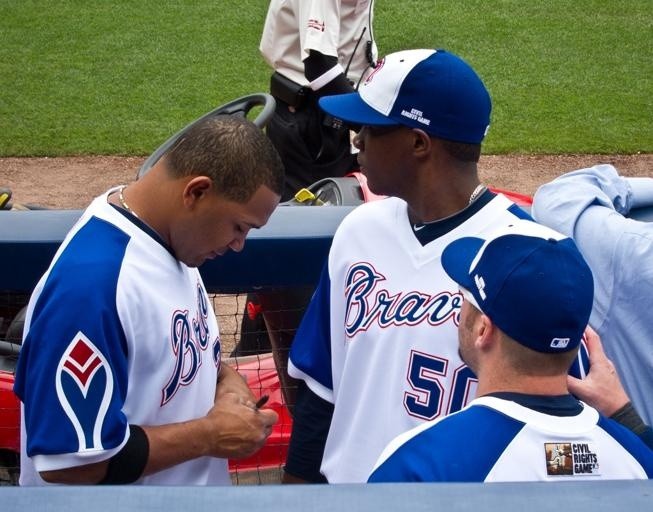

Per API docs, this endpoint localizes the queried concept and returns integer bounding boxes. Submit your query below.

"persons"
[256,0,376,203]
[562,323,652,452]
[13,117,284,490]
[276,48,598,490]
[362,220,652,481]
[530,161,653,429]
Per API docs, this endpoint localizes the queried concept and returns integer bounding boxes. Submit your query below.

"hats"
[441,233,595,353]
[318,48,492,145]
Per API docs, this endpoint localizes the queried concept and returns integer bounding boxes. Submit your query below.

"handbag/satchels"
[270,71,309,110]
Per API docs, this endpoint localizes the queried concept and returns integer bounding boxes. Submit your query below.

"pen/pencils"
[252,395,268,411]
